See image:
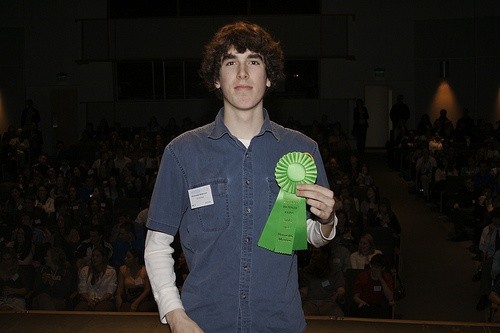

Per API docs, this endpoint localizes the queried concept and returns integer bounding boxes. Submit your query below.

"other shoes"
[476,295,491,310]
[471,255,483,261]
[472,271,482,281]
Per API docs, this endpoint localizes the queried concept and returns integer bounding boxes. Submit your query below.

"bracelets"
[317,210,334,225]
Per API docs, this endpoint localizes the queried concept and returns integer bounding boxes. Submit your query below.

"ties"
[494,229,500,254]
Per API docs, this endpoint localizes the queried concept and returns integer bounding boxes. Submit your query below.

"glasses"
[370,264,381,269]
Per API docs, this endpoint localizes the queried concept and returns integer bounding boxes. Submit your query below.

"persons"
[0,94,500,322]
[144,22,340,333]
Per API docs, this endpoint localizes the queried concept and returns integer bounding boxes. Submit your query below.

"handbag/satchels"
[392,285,407,304]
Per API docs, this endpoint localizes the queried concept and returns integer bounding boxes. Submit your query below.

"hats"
[370,253,387,266]
